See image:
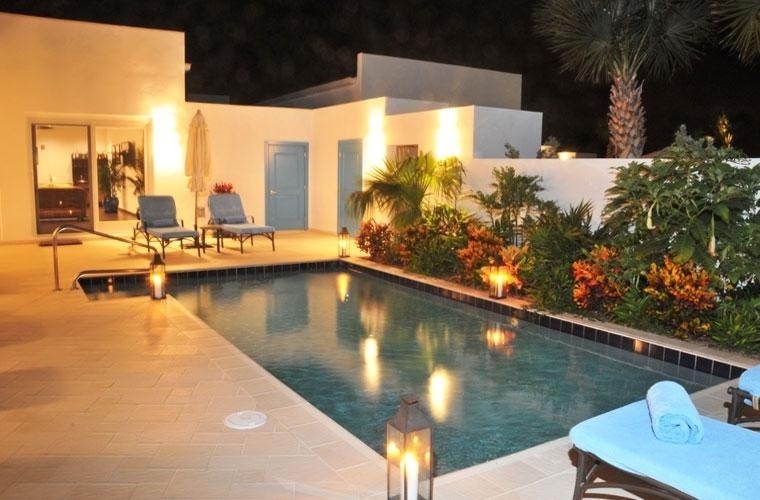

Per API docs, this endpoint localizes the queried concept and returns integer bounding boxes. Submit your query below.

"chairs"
[131,192,276,254]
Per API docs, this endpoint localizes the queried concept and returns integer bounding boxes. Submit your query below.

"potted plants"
[97,154,127,213]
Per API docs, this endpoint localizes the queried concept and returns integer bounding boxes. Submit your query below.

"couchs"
[38,186,88,223]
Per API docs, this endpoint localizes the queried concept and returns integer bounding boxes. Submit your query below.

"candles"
[400,450,421,500]
[153,274,163,298]
[496,274,504,297]
[341,240,348,256]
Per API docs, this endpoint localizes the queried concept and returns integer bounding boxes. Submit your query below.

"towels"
[646,379,704,446]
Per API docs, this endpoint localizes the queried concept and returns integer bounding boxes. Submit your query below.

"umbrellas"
[184,110,211,243]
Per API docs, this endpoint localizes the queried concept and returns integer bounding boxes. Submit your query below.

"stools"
[566,397,757,498]
